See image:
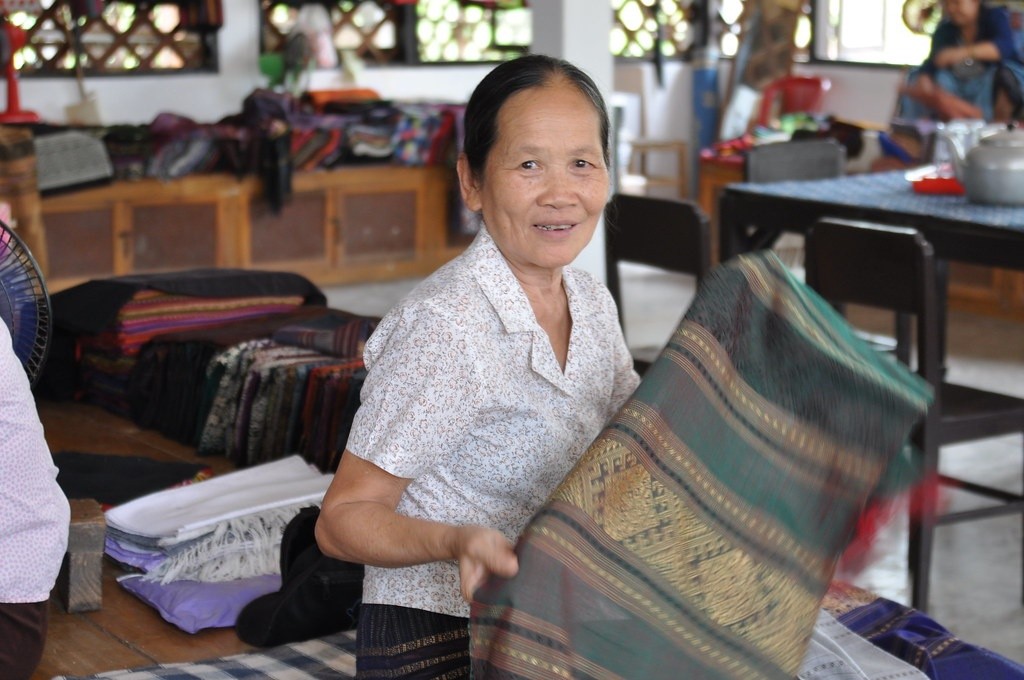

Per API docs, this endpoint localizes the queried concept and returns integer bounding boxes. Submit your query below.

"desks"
[719,168,1024,382]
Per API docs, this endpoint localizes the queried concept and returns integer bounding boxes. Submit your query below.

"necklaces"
[955,36,978,66]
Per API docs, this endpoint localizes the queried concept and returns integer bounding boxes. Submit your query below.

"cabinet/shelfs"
[0,166,478,295]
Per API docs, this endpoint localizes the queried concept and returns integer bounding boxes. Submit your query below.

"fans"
[1,219,54,392]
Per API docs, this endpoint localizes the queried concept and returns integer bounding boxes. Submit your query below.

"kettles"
[940,120,1023,204]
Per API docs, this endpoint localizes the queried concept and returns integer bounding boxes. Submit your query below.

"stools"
[629,139,691,200]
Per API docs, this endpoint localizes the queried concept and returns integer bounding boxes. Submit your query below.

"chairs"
[697,73,831,223]
[604,194,713,379]
[743,142,846,183]
[804,216,1024,614]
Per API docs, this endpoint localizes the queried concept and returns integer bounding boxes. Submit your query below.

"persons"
[899,0,1024,120]
[0,316,71,680]
[314,54,642,680]
